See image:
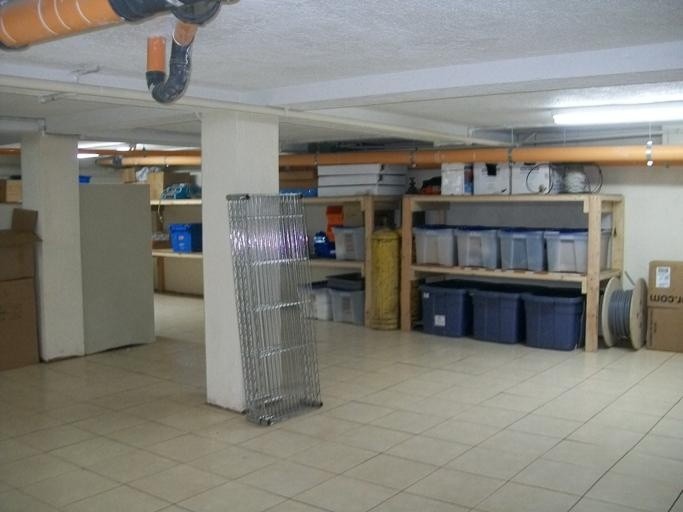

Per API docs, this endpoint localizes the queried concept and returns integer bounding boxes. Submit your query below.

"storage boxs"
[329,201,367,261]
[411,217,613,273]
[439,160,572,194]
[168,222,203,254]
[146,172,192,200]
[0,204,42,368]
[646,262,683,356]
[419,277,589,350]
[277,161,406,198]
[298,279,365,325]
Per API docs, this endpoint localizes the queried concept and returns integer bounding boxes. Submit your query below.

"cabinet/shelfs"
[278,194,394,327]
[149,199,204,260]
[401,192,626,351]
